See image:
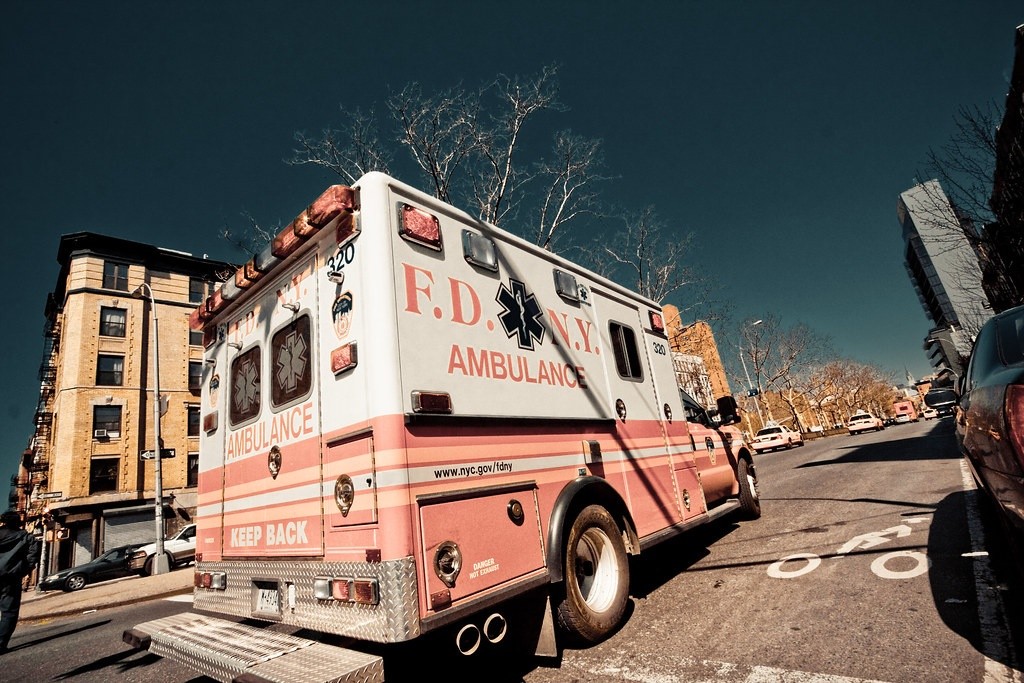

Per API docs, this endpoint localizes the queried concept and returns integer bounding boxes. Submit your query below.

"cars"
[894,412,913,424]
[923,409,938,420]
[125,520,197,577]
[40,542,162,594]
[848,412,894,436]
[750,422,804,454]
[921,305,1024,552]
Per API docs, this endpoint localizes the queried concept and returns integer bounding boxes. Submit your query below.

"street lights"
[131,281,171,574]
[936,367,958,379]
[738,319,765,430]
[926,337,962,359]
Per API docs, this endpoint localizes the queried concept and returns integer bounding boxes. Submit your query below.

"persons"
[0,511,38,655]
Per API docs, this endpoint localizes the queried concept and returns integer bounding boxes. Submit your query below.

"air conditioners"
[95,430,106,436]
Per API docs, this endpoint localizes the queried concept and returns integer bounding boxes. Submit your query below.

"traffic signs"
[140,448,175,461]
[36,491,62,499]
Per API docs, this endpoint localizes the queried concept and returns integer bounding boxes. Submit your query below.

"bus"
[892,401,920,422]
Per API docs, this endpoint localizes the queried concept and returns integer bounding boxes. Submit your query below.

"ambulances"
[124,170,761,683]
